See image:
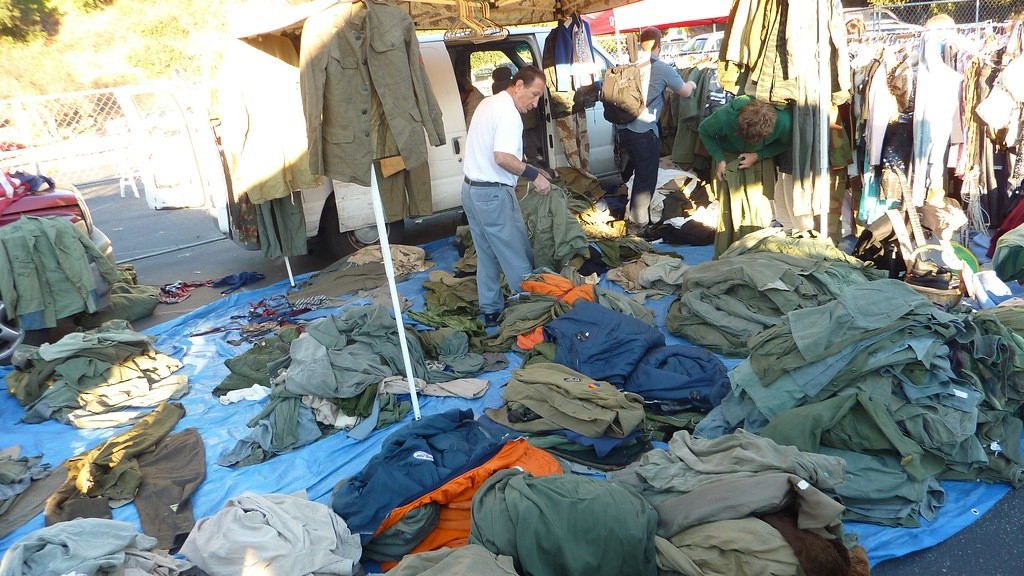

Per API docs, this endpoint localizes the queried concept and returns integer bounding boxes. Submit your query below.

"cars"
[845,7,922,33]
[608,31,725,67]
[0,178,116,367]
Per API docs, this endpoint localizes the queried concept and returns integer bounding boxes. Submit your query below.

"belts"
[463,174,512,189]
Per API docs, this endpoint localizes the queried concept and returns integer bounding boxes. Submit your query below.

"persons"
[927,14,955,28]
[456,80,483,131]
[844,17,867,34]
[461,67,555,327]
[696,96,794,253]
[618,27,698,226]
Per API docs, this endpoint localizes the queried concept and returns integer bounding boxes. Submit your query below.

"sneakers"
[484,311,502,327]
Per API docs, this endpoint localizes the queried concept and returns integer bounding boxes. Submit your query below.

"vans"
[210,27,636,262]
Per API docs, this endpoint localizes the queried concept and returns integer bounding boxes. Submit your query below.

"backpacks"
[601,58,661,125]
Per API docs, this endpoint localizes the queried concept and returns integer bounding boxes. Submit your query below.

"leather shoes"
[911,259,948,279]
[906,270,952,290]
